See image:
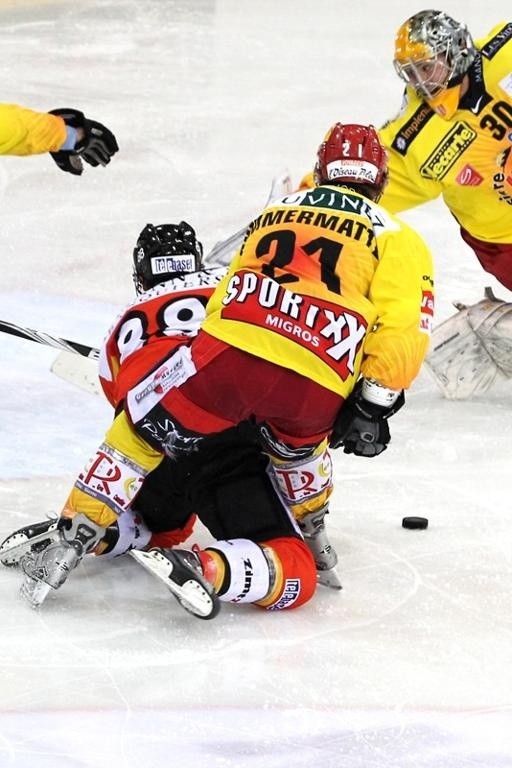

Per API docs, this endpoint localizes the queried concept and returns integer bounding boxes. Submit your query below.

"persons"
[300,11,512,383]
[0,98,119,176]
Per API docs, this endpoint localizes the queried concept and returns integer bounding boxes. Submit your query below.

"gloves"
[133,221,203,291]
[329,378,405,457]
[48,108,120,176]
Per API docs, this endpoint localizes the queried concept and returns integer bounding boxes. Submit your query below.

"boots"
[0,519,85,589]
[147,546,221,620]
[297,500,337,570]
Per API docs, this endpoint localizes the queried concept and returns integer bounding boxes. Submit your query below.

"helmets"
[313,122,390,191]
[393,9,477,75]
